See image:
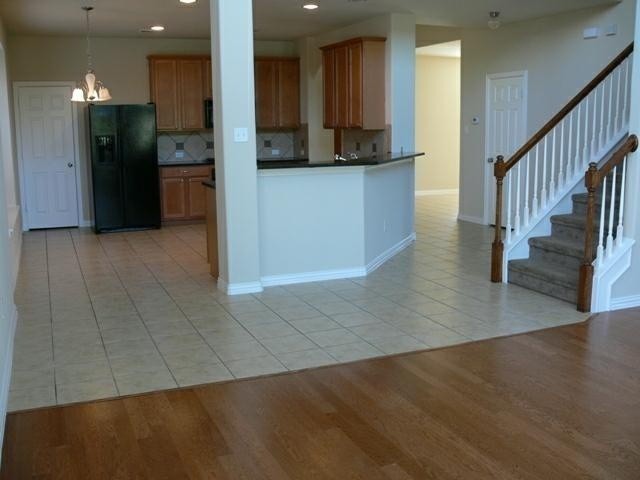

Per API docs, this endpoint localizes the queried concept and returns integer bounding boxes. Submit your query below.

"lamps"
[70,6,112,102]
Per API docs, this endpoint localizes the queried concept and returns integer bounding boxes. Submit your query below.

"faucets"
[335,154,347,161]
[350,152,359,159]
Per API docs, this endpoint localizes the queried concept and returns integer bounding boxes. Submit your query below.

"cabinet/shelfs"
[319,35,386,131]
[254,56,299,130]
[145,54,205,133]
[159,164,211,226]
